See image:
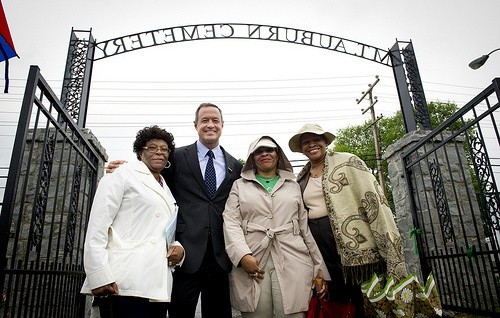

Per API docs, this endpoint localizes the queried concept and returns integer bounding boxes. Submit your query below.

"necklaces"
[312,164,323,176]
[259,177,273,183]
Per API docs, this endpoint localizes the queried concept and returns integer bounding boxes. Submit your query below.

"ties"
[205,151,217,198]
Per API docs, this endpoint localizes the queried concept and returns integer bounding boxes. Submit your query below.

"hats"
[288,123,336,152]
[251,138,277,153]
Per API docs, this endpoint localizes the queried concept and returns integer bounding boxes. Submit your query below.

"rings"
[169,261,172,266]
[250,272,257,276]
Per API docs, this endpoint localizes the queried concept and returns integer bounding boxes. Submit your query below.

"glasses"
[253,147,277,155]
[143,146,172,154]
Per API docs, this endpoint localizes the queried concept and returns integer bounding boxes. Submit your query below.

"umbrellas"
[361,272,442,318]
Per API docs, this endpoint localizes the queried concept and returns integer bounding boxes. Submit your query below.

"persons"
[80,125,186,318]
[222,135,331,318]
[289,124,411,318]
[106,103,242,318]
